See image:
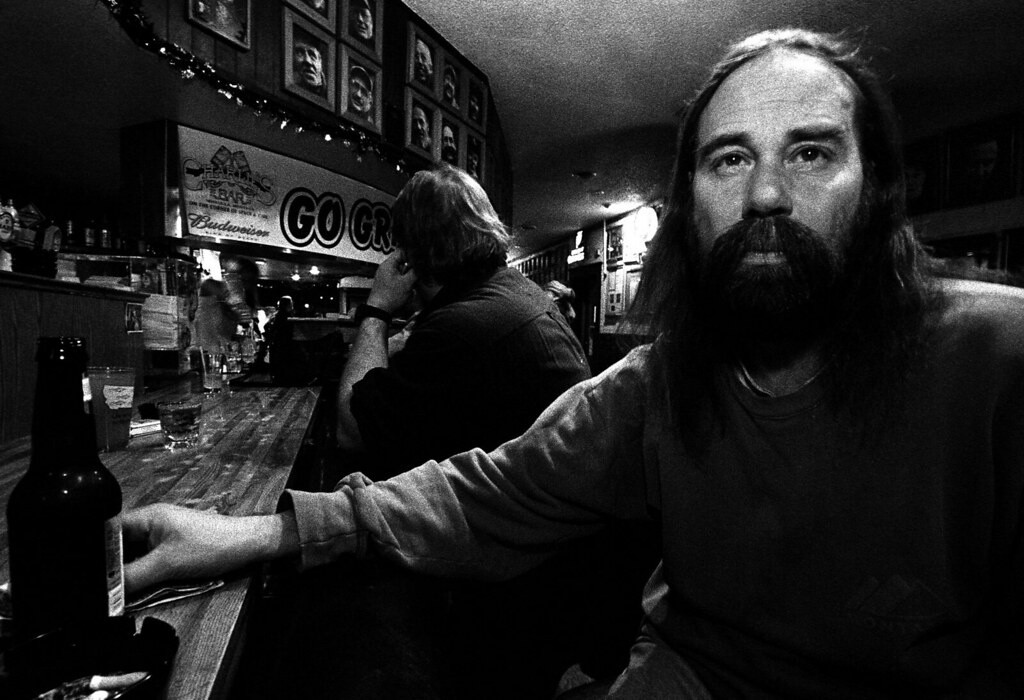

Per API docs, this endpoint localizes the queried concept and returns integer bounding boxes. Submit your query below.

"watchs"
[355,303,392,326]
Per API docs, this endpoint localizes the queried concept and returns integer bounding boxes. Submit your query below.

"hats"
[361,0,370,10]
[349,65,373,92]
[445,65,457,83]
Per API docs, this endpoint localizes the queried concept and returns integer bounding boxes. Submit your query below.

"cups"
[154,393,203,440]
[88,366,136,452]
[199,345,226,392]
[224,342,242,374]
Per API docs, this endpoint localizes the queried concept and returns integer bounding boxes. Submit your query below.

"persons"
[348,66,374,124]
[443,126,458,165]
[252,307,271,349]
[470,87,482,122]
[266,296,294,380]
[414,39,435,88]
[121,28,1024,700]
[443,66,460,109]
[413,107,431,151]
[284,166,610,697]
[467,137,480,177]
[197,0,243,38]
[349,0,375,48]
[294,30,327,98]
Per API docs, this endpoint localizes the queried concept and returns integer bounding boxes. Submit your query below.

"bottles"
[5,336,130,678]
[0,198,158,265]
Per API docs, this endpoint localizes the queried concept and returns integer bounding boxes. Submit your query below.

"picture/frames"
[403,86,438,164]
[282,7,337,111]
[338,0,383,67]
[406,21,438,103]
[337,43,382,137]
[283,0,337,37]
[462,123,485,187]
[437,109,462,171]
[440,49,464,121]
[466,70,486,135]
[187,0,251,51]
